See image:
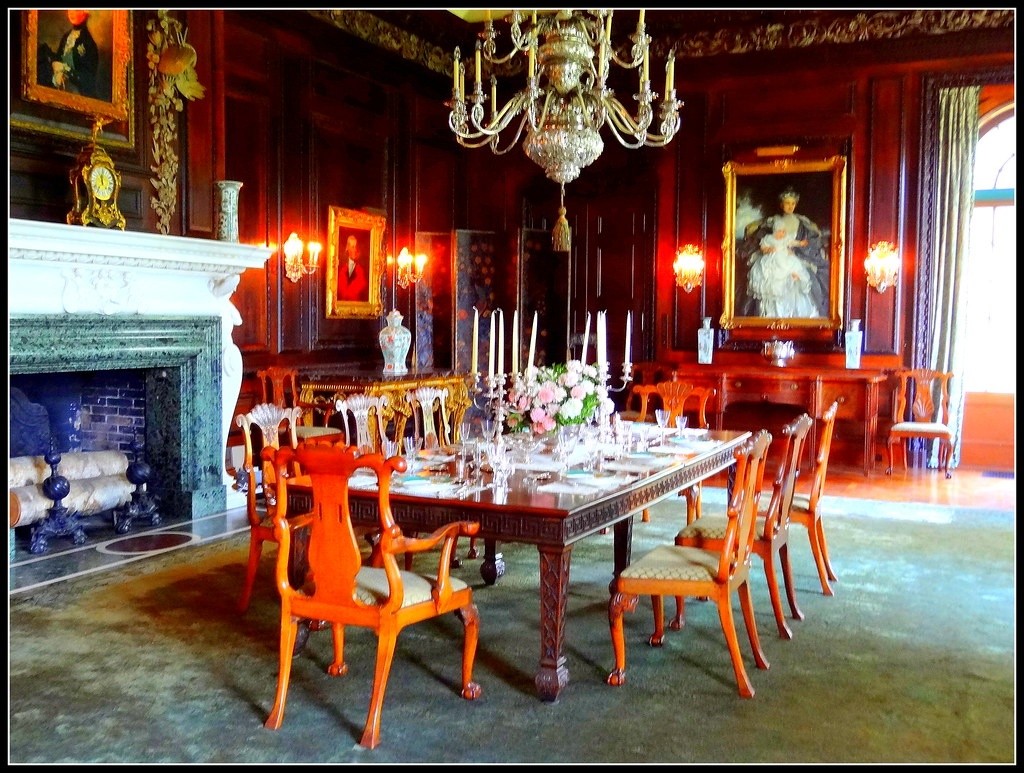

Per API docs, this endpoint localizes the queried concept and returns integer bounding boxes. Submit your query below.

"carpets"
[8,487,1015,764]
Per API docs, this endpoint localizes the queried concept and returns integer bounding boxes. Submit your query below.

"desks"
[267,420,752,703]
[299,377,473,454]
[671,361,889,478]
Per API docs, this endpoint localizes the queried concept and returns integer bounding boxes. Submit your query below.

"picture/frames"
[21,10,131,122]
[325,205,386,319]
[718,154,847,330]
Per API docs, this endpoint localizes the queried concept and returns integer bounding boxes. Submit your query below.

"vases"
[697,317,714,364]
[844,319,863,369]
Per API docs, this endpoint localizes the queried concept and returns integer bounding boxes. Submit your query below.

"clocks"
[65,112,125,231]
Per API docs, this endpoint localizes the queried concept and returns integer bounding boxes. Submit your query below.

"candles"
[470,306,632,378]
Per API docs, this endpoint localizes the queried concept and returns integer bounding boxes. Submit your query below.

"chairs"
[234,357,837,749]
[885,371,954,479]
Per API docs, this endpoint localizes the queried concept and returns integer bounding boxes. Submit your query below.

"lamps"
[284,231,323,283]
[397,246,427,289]
[673,245,704,293]
[862,240,901,293]
[449,10,684,251]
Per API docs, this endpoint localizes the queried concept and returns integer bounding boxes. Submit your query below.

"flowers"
[506,362,620,440]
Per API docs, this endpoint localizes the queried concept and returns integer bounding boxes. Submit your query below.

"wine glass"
[382,410,689,491]
